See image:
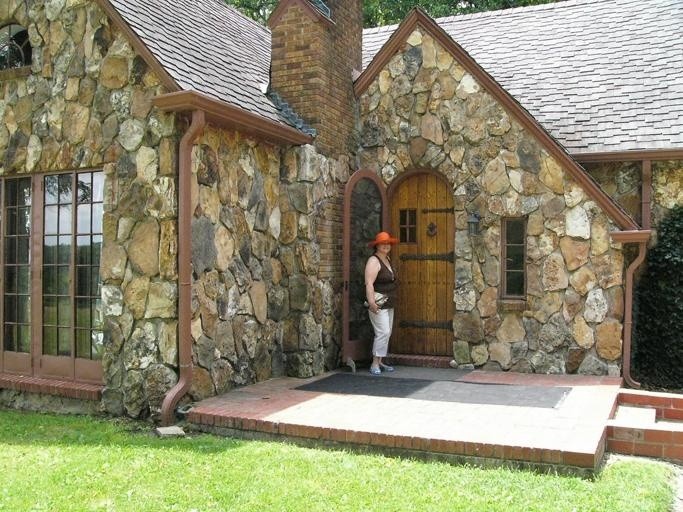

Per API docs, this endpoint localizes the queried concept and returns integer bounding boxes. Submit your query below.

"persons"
[361,228,402,376]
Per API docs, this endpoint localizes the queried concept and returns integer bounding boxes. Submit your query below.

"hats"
[368,232,399,248]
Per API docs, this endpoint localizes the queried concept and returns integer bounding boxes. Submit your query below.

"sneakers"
[369,364,382,375]
[380,362,394,372]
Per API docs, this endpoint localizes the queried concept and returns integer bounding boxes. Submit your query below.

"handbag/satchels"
[363,292,388,312]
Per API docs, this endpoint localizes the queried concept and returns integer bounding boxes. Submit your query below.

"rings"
[375,310,379,313]
